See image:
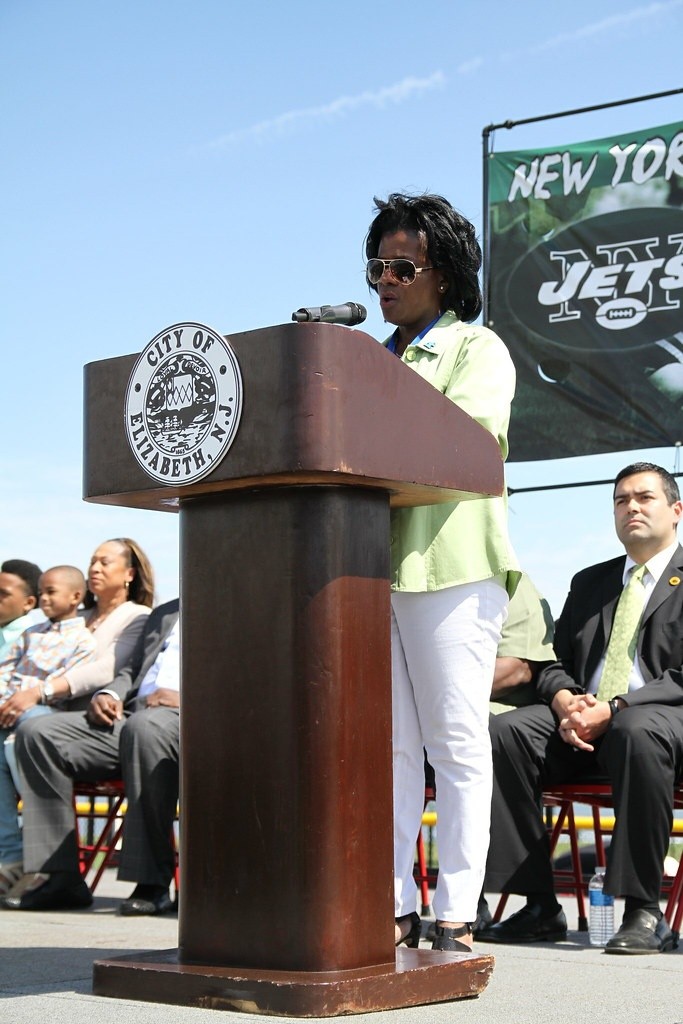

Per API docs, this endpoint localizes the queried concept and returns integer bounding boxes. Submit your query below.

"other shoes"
[426,895,492,940]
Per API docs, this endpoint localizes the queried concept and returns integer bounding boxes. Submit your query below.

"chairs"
[73,781,683,949]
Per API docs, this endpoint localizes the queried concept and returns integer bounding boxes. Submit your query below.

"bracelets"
[39,684,46,705]
[609,701,619,718]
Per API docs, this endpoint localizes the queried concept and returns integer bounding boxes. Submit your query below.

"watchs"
[43,680,53,701]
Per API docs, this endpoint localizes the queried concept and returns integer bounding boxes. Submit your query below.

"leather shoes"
[5,877,94,911]
[473,902,567,945]
[605,908,675,954]
[118,882,170,913]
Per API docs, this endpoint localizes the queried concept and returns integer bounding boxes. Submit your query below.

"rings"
[8,709,17,715]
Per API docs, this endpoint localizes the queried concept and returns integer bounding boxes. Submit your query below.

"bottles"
[588,867,614,946]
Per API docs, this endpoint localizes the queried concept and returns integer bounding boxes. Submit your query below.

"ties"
[596,563,648,703]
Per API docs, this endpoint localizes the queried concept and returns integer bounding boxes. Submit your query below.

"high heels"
[433,923,472,954]
[390,911,422,950]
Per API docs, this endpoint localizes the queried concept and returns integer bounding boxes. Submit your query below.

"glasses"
[366,258,433,287]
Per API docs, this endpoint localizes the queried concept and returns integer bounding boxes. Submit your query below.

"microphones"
[292,302,368,326]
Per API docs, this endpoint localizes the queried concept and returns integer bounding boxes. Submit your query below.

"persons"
[363,186,516,951]
[471,462,683,953]
[423,573,557,933]
[0,536,181,915]
